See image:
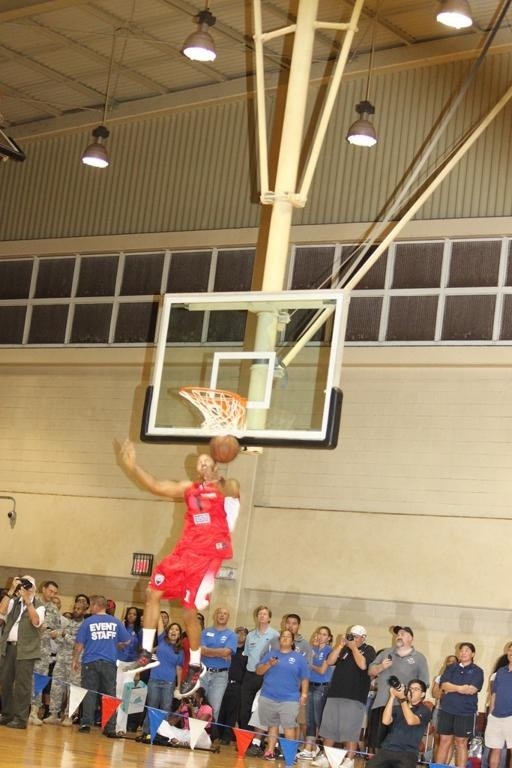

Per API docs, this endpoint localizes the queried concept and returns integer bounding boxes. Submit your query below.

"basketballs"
[210,436,238,464]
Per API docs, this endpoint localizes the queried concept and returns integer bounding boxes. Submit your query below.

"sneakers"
[246,744,263,756]
[102,730,117,737]
[179,661,207,697]
[118,731,126,737]
[77,724,91,733]
[310,755,329,768]
[339,757,355,768]
[264,750,276,760]
[0,713,26,730]
[296,745,320,760]
[121,649,160,674]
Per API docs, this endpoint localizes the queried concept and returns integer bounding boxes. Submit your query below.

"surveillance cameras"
[7,511,16,519]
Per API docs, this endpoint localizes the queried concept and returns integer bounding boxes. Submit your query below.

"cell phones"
[272,655,279,659]
[388,654,392,661]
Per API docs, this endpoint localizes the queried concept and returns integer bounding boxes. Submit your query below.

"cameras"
[13,578,32,591]
[183,696,197,704]
[387,675,412,701]
[347,634,353,642]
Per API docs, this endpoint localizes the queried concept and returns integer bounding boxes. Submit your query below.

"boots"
[29,704,42,726]
[44,711,73,726]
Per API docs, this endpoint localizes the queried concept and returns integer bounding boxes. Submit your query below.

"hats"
[393,626,413,638]
[22,574,36,590]
[350,625,369,639]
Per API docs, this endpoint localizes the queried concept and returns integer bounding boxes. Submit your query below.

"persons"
[120,437,242,700]
[0,576,512,767]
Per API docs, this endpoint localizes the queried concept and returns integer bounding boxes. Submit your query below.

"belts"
[207,667,228,673]
[309,682,328,686]
[229,680,237,684]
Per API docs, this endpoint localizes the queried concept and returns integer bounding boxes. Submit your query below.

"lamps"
[79,28,120,167]
[347,0,386,147]
[178,0,217,63]
[434,0,473,29]
